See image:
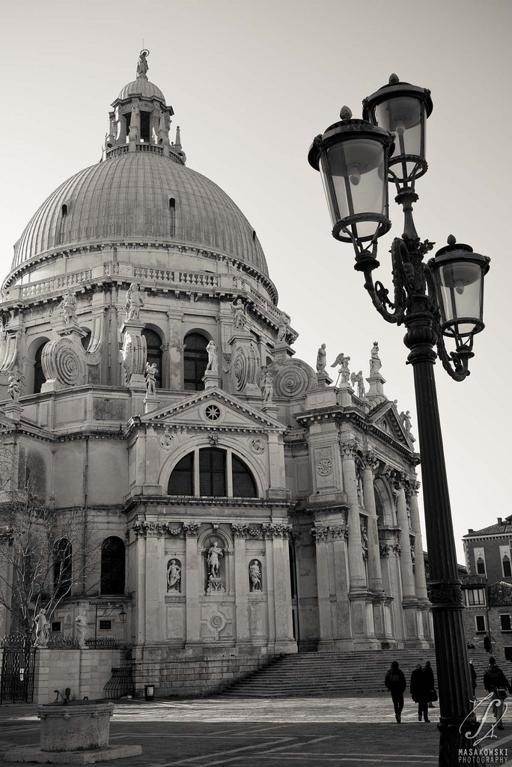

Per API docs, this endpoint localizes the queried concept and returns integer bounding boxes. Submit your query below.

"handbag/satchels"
[496,688,507,699]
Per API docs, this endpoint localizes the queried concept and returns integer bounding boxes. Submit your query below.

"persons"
[250,561,261,591]
[393,399,413,433]
[207,542,223,578]
[315,341,383,399]
[205,340,218,371]
[167,560,181,590]
[74,610,88,646]
[34,609,50,647]
[410,661,438,723]
[384,660,406,725]
[483,657,512,731]
[145,362,158,394]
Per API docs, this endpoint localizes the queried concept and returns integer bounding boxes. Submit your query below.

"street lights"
[307,72,482,764]
